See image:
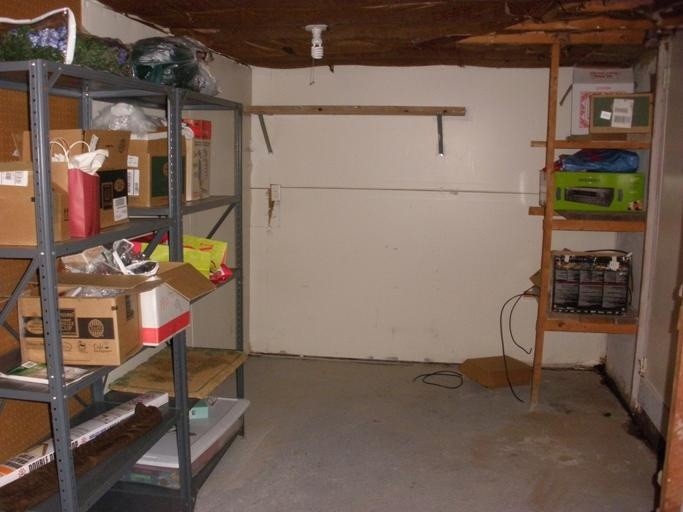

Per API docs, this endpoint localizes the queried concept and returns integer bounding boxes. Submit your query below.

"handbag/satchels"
[46,134,109,240]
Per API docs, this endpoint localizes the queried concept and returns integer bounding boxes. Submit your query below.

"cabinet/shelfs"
[527,41,645,413]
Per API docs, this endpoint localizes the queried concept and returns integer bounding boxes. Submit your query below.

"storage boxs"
[538,169,644,212]
[565,61,633,136]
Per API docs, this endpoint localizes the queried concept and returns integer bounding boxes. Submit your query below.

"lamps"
[305,21,327,60]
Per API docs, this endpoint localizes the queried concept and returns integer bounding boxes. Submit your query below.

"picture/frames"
[587,95,652,133]
